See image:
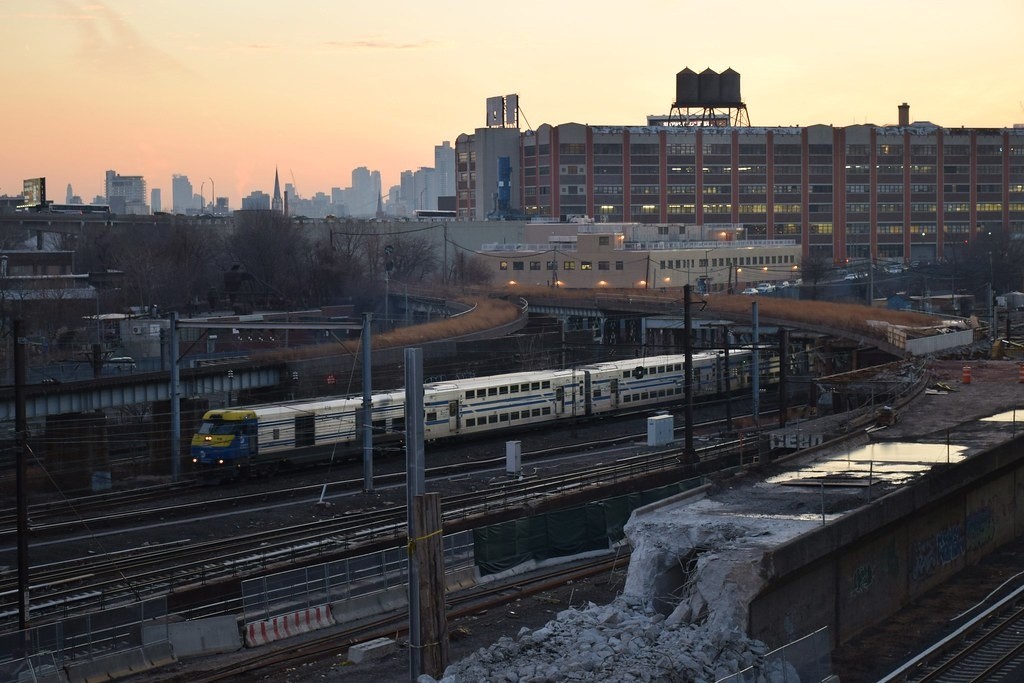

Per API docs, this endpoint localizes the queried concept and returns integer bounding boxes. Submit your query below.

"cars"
[844,272,867,282]
[742,288,759,295]
[155,212,213,217]
[291,214,409,222]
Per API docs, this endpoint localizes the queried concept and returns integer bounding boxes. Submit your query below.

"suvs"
[102,357,137,376]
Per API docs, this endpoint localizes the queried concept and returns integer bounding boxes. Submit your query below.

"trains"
[191,344,780,481]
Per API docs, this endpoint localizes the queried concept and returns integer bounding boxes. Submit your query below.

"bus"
[49,203,111,214]
[413,210,457,222]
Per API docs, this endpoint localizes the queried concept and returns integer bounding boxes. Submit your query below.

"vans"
[775,279,803,290]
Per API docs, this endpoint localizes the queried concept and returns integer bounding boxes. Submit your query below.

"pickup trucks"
[756,283,776,294]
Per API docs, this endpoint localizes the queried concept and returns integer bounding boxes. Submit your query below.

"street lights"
[201,182,204,213]
[210,178,214,215]
[421,188,427,210]
